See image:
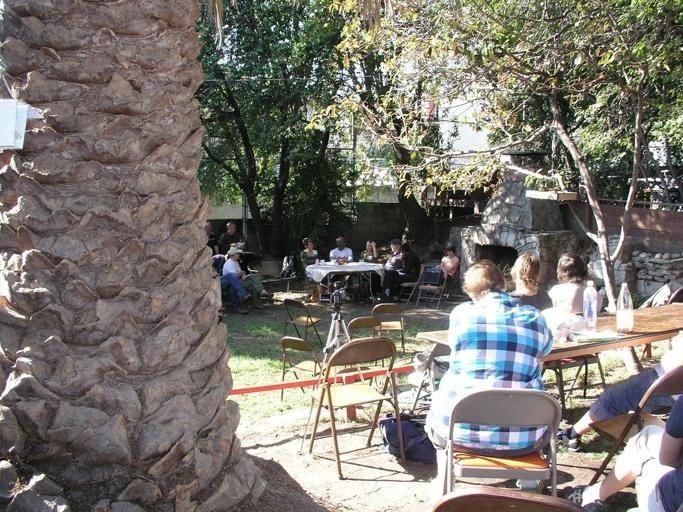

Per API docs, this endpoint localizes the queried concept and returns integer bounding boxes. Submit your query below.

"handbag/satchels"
[413,352,450,381]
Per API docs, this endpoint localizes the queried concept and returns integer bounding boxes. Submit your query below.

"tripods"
[299,308,367,455]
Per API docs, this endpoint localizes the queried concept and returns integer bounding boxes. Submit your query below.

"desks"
[411,303,683,447]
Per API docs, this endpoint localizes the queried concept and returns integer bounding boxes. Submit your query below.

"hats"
[390,239,401,244]
[228,247,243,255]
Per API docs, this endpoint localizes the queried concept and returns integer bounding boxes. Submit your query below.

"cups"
[616,282,634,332]
[583,280,598,327]
[320,260,325,266]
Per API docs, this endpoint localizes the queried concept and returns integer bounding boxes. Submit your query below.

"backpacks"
[381,409,436,464]
[280,255,306,278]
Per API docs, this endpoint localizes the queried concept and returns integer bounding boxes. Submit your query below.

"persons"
[438,247,460,296]
[548,252,603,315]
[300,238,327,295]
[557,330,683,452]
[206,220,274,314]
[330,237,353,291]
[422,260,553,489]
[508,253,553,313]
[562,394,683,512]
[358,238,420,303]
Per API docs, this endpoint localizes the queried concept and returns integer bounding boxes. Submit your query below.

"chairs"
[440,391,562,495]
[281,297,323,346]
[429,485,582,511]
[428,259,461,304]
[248,257,296,294]
[415,267,449,310]
[279,334,327,398]
[347,317,386,368]
[540,330,607,398]
[390,263,424,303]
[586,363,683,486]
[308,339,409,480]
[371,303,407,357]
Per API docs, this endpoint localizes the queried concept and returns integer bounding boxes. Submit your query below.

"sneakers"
[556,427,583,452]
[563,485,616,511]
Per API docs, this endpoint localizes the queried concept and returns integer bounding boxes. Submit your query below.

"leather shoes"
[260,291,274,299]
[240,294,251,303]
[238,309,249,313]
[253,303,268,309]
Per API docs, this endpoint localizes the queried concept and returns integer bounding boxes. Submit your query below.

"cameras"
[320,280,346,306]
